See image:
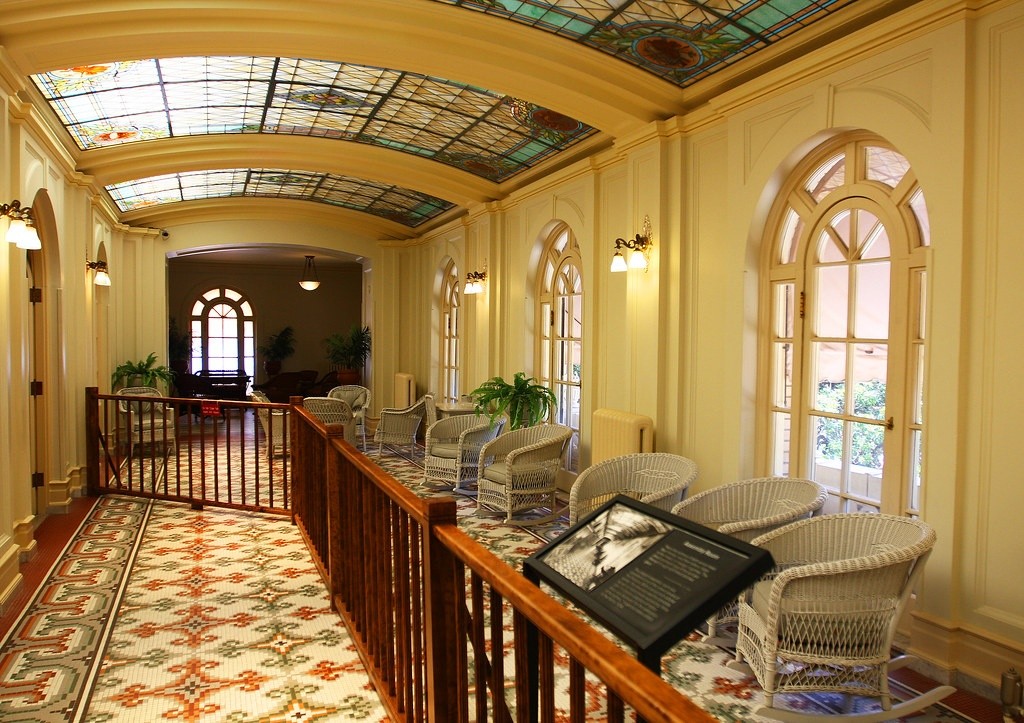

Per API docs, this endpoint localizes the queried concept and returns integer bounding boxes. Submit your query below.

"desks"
[435,402,487,419]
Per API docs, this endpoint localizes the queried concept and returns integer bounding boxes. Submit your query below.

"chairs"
[190,378,227,422]
[671,477,828,647]
[570,452,699,529]
[302,385,508,492]
[117,387,178,455]
[735,509,957,722]
[475,423,573,525]
[251,390,290,458]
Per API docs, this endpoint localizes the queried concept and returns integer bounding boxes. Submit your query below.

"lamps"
[297,255,321,291]
[85,259,112,285]
[0,200,42,250]
[464,270,486,295]
[611,216,652,272]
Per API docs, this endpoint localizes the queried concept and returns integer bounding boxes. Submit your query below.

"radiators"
[591,408,654,512]
[394,373,416,409]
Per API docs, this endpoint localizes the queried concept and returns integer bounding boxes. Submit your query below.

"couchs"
[175,369,360,414]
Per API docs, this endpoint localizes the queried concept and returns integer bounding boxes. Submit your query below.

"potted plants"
[111,352,176,391]
[467,371,559,430]
[259,326,297,376]
[322,327,373,385]
[169,319,192,373]
